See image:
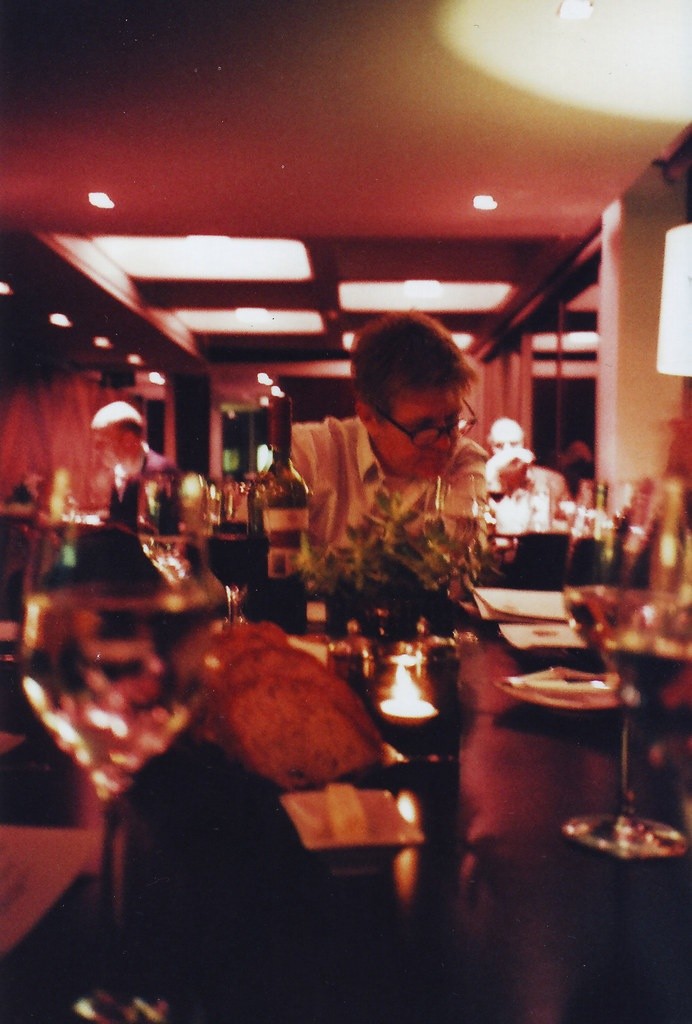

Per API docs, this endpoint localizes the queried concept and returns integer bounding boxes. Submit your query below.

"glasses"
[363,394,477,449]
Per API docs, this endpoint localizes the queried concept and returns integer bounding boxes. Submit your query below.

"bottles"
[247,394,311,637]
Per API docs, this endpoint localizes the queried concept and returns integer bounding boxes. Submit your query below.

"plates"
[489,665,624,711]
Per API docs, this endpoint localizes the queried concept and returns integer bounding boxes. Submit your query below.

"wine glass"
[19,467,230,1024]
[561,478,692,861]
[426,474,478,604]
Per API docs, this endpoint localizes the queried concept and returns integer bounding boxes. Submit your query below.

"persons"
[484,412,573,534]
[91,402,180,533]
[289,311,496,593]
[268,450,305,507]
